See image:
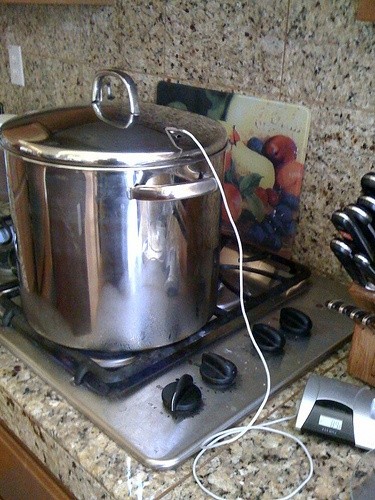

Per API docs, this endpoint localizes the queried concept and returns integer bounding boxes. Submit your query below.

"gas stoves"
[0,210,359,471]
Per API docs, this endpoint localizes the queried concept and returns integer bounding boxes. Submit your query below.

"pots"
[0,71,229,356]
